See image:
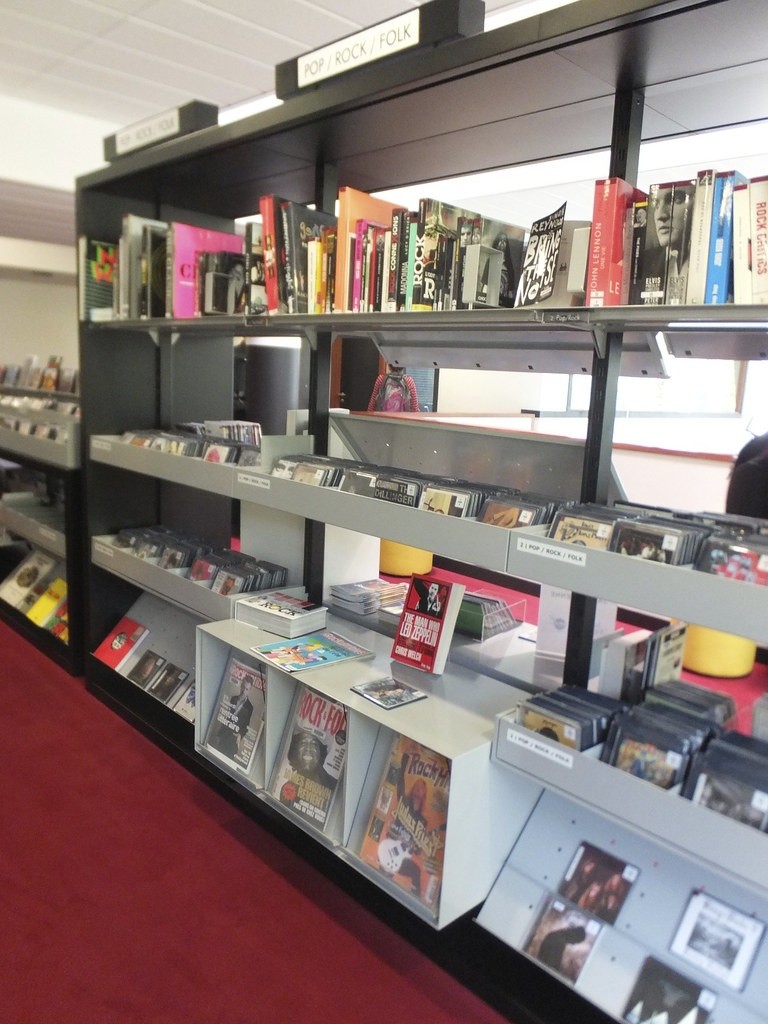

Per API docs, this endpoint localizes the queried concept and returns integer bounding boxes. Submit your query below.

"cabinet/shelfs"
[0,386,82,677]
[75,1,768,1024]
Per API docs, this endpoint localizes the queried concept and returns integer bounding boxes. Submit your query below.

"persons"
[379,741,440,900]
[635,180,698,306]
[460,223,473,248]
[472,225,481,244]
[480,231,516,300]
[216,674,255,758]
[367,360,419,415]
[560,855,629,920]
[279,727,337,830]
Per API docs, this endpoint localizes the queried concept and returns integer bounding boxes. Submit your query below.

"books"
[0,547,450,921]
[78,169,768,323]
[391,572,466,678]
